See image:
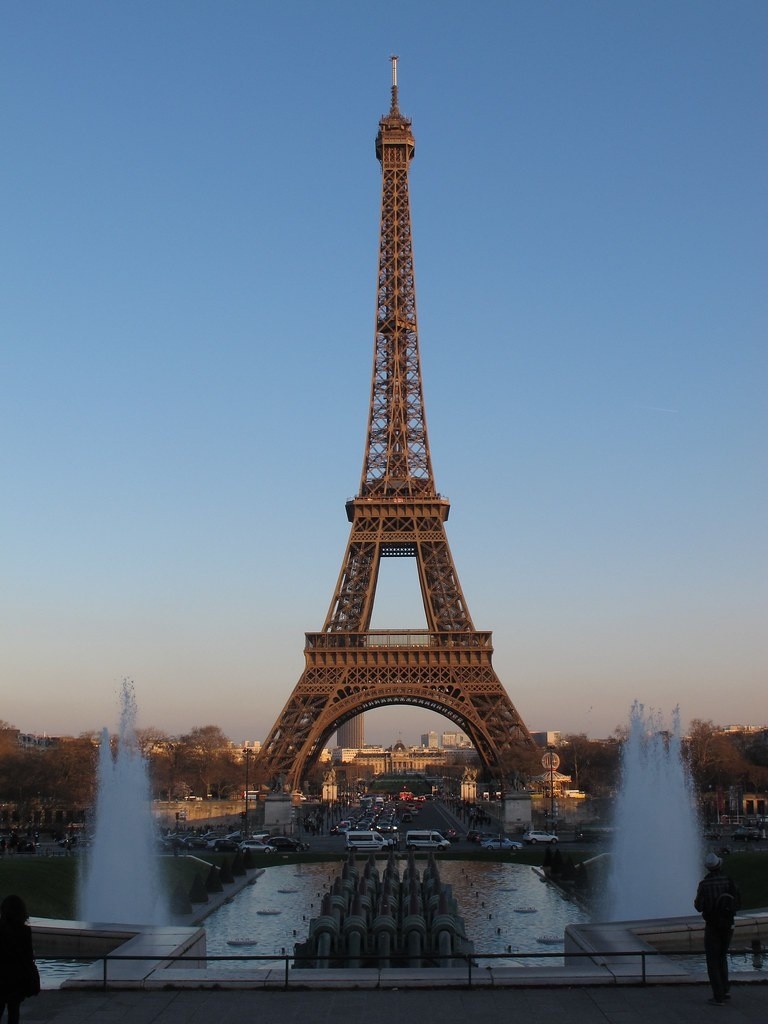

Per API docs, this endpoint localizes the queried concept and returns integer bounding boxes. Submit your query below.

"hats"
[704,853,722,871]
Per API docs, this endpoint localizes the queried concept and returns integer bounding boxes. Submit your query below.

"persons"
[542,844,588,892]
[0,895,40,1024]
[694,853,742,1005]
[303,812,325,836]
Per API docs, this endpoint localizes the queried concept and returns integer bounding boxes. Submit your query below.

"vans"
[291,793,306,801]
[405,830,452,850]
[344,830,389,851]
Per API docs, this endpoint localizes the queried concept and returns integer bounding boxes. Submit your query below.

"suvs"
[523,831,559,845]
[731,827,762,842]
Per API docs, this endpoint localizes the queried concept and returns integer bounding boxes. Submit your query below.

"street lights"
[545,745,555,819]
[243,749,253,814]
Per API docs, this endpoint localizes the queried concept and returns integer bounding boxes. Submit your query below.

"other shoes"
[720,993,731,1001]
[707,997,725,1006]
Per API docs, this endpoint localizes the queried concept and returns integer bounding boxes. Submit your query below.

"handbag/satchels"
[25,962,40,998]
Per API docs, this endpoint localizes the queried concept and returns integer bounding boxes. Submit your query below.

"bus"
[375,797,384,807]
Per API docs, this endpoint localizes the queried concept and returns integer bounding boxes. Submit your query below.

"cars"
[0,835,35,853]
[159,829,310,854]
[330,793,437,835]
[481,838,524,851]
[703,832,721,841]
[59,833,98,849]
[415,828,499,845]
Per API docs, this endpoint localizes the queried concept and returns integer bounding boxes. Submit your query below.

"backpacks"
[711,877,735,933]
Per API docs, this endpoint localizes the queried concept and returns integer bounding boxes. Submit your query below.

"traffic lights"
[243,812,246,819]
